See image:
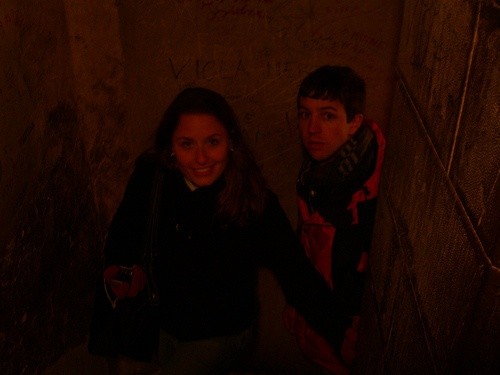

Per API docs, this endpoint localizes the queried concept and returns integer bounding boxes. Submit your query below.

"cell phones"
[111,267,133,289]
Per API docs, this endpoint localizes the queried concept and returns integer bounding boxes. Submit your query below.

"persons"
[101,85,364,374]
[281,64,387,374]
